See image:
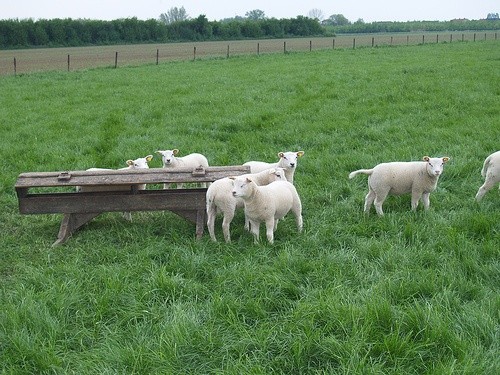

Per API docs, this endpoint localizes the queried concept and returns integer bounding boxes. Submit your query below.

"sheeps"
[474,151,500,207]
[229,176,303,244]
[85,154,153,222]
[206,151,305,244]
[154,149,213,189]
[348,156,450,217]
